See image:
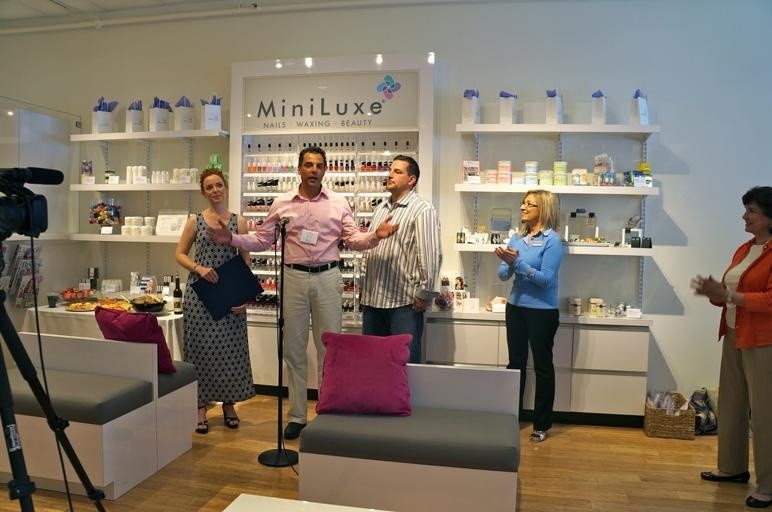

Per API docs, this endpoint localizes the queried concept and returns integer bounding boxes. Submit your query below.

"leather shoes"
[700,471,750,483]
[745,496,772,508]
[285,420,306,439]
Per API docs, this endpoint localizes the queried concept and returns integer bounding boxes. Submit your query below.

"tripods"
[0,249,107,512]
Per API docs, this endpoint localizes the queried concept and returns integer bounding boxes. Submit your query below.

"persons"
[690,185,771,512]
[359,152,442,367]
[171,165,254,434]
[203,144,403,440]
[495,188,564,444]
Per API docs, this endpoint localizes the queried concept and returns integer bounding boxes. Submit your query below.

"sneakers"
[530,429,547,440]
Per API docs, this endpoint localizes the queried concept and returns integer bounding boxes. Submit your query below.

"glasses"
[519,201,537,209]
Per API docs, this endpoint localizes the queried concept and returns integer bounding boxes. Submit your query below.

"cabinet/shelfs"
[239,124,418,322]
[452,123,662,258]
[67,128,230,243]
[421,303,654,434]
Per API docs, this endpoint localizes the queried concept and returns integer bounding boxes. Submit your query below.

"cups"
[47,296,58,308]
[121,225,132,237]
[131,225,142,237]
[141,226,152,237]
[125,217,133,225]
[145,217,156,235]
[133,216,144,226]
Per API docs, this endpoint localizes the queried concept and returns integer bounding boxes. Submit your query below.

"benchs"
[299,362,523,512]
[1,331,200,501]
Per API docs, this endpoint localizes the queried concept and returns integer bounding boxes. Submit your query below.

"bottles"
[455,225,503,245]
[249,255,281,273]
[151,168,170,185]
[339,258,362,276]
[589,295,628,318]
[248,294,279,311]
[208,154,224,171]
[342,299,354,313]
[246,142,297,174]
[173,168,199,185]
[254,274,280,292]
[173,276,183,314]
[155,274,174,296]
[571,151,653,188]
[103,169,117,184]
[338,241,360,255]
[245,216,266,231]
[79,158,93,177]
[356,175,387,193]
[346,196,389,213]
[271,238,282,252]
[322,175,356,194]
[125,160,148,185]
[360,139,412,174]
[343,280,357,295]
[244,176,299,195]
[562,208,610,247]
[242,196,274,212]
[565,296,582,317]
[358,220,371,234]
[101,279,123,293]
[301,141,355,173]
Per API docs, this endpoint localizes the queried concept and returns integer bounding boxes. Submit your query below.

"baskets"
[644,391,696,438]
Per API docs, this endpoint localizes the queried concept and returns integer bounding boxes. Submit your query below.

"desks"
[21,300,185,360]
[221,490,386,512]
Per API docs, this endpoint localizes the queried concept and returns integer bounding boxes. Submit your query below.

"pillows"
[318,331,414,417]
[93,306,178,376]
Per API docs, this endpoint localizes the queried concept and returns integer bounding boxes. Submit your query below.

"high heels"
[222,401,240,428]
[195,405,208,434]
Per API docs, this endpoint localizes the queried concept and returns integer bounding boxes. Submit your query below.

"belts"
[283,262,339,273]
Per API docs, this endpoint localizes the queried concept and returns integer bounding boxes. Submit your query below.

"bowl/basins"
[130,298,167,312]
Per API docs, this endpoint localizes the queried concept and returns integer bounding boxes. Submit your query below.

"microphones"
[276,217,289,227]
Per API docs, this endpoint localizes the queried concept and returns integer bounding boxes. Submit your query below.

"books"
[1,241,42,309]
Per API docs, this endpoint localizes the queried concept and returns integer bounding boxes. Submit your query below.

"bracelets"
[192,263,202,272]
[724,290,737,306]
